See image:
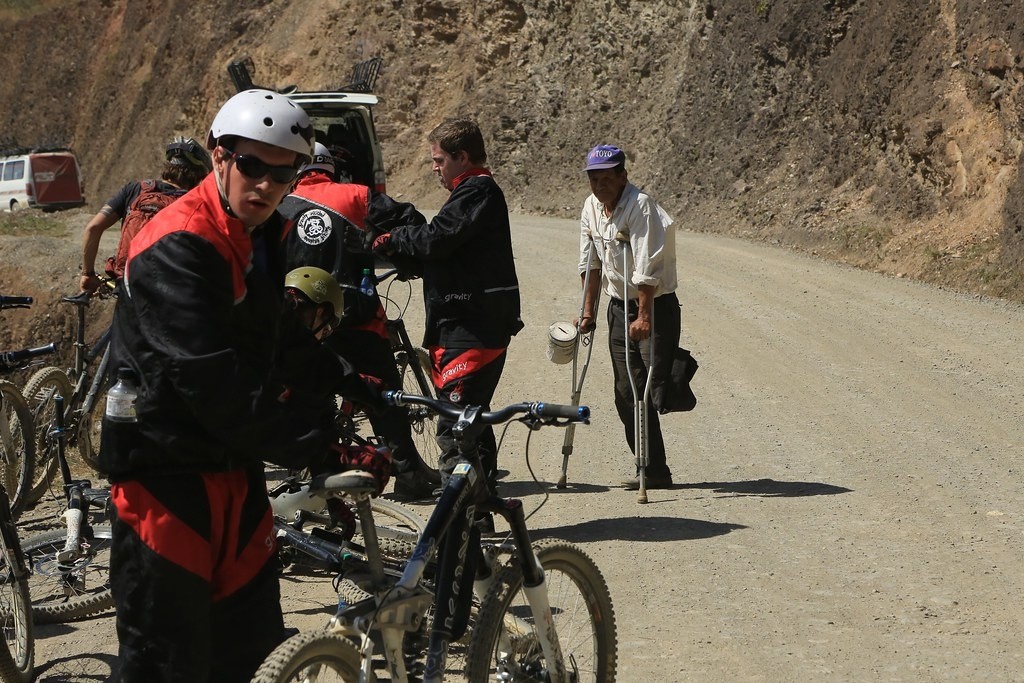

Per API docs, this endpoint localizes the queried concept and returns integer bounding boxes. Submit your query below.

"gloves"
[344,217,398,261]
[308,445,391,499]
[340,373,389,414]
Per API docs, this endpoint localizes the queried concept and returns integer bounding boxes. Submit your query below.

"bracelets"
[82,272,95,275]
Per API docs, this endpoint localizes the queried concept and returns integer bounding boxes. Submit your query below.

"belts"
[610,294,670,308]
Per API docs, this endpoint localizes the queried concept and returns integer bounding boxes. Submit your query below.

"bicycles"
[1,262,619,683]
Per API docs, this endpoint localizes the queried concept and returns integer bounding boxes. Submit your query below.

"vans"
[279,90,389,192]
[0,147,86,212]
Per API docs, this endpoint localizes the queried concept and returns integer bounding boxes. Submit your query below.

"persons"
[79,136,214,295]
[286,267,343,342]
[572,144,699,490]
[99,89,398,683]
[280,140,439,498]
[371,120,524,581]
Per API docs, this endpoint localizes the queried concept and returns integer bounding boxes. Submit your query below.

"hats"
[582,144,626,172]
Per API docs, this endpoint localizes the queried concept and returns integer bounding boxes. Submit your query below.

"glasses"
[223,148,298,184]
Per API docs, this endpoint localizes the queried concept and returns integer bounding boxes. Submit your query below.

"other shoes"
[394,465,444,504]
[622,466,673,487]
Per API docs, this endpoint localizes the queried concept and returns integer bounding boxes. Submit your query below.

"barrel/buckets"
[548,321,577,364]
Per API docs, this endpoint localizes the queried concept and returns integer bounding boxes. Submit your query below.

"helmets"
[166,136,213,175]
[285,267,344,329]
[298,141,335,174]
[205,89,316,174]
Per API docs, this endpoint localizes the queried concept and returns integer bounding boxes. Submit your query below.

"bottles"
[359,269,374,297]
[105,368,139,422]
